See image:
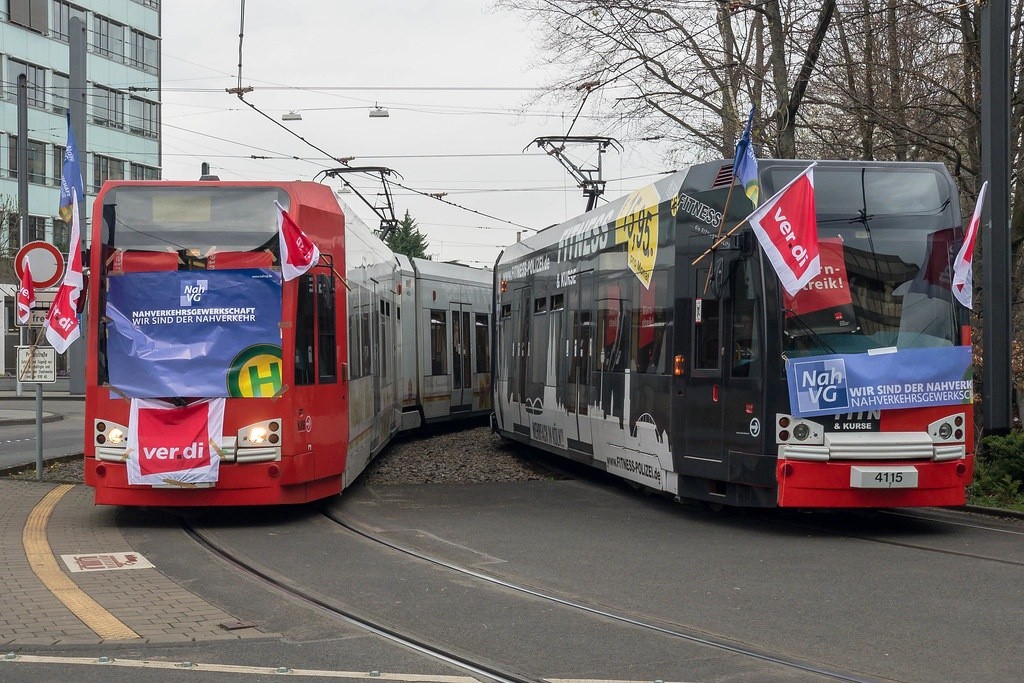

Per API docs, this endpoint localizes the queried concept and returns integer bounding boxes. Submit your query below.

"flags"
[733,107,760,211]
[43,190,84,354]
[59,113,83,223]
[908,226,964,304]
[747,165,821,298]
[274,200,320,282]
[18,262,36,324]
[953,181,989,310]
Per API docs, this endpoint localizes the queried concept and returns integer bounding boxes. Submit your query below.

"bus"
[487,133,977,525]
[75,165,498,509]
